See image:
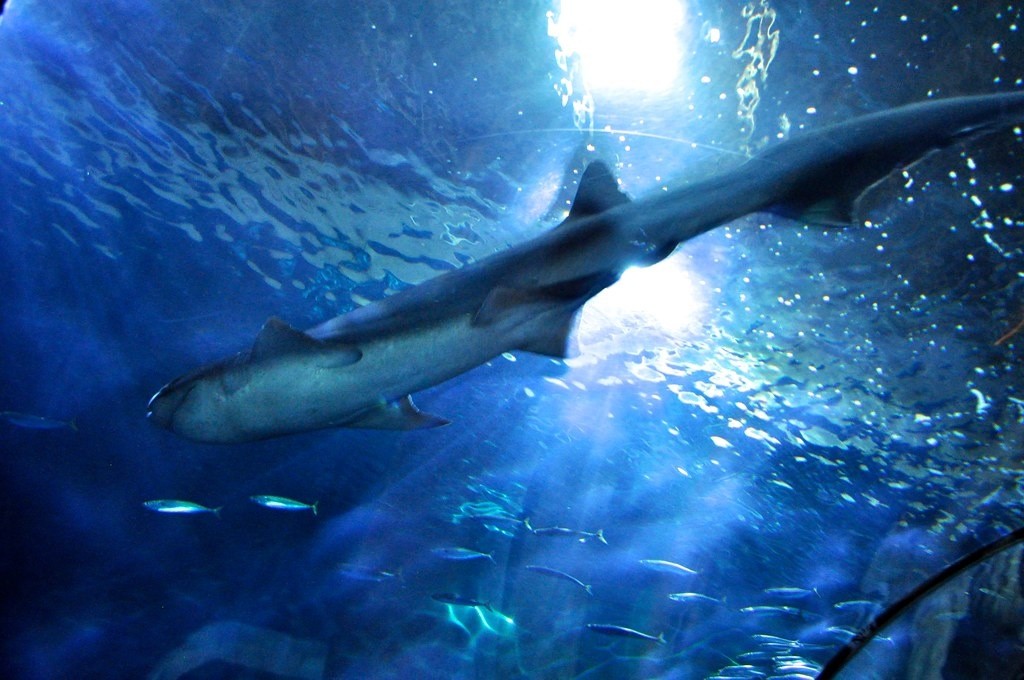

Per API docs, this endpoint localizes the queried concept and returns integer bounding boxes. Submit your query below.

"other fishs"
[141,500,225,521]
[249,494,321,517]
[430,590,496,614]
[738,587,896,650]
[0,0,1023,626]
[336,562,403,584]
[532,526,608,544]
[584,620,667,649]
[1,410,77,434]
[430,546,497,563]
[706,632,837,680]
[525,563,593,597]
[667,592,729,607]
[638,557,703,577]
